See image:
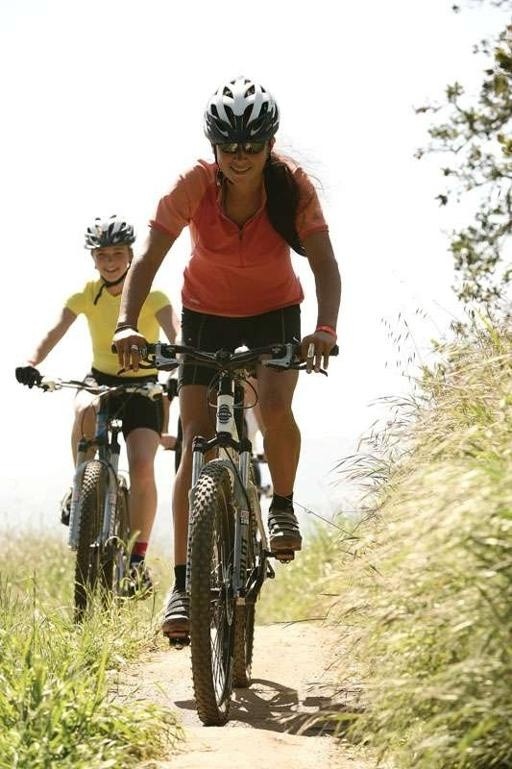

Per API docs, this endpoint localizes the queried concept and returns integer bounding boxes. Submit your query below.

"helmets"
[202,76,279,145]
[83,214,136,250]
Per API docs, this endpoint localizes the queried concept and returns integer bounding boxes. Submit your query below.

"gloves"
[15,367,44,390]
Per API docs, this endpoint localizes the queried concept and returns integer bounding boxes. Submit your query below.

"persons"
[14,221,183,594]
[114,78,341,633]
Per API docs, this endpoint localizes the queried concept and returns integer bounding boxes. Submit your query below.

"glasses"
[218,142,266,154]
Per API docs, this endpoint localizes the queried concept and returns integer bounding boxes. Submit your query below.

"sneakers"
[121,566,153,601]
[162,592,191,647]
[267,507,301,563]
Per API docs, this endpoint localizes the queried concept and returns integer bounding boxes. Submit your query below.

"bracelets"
[27,359,36,367]
[316,325,338,339]
[116,326,139,332]
